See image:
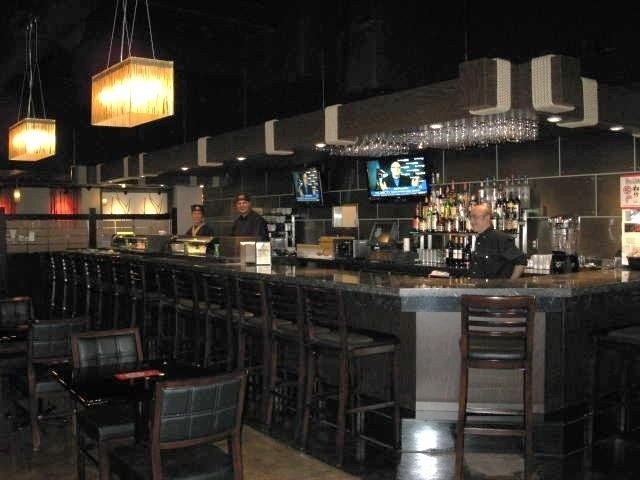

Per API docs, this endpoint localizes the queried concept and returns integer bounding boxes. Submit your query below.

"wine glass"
[407,120,539,151]
[327,133,410,158]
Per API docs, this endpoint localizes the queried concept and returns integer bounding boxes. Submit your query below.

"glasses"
[469,214,485,220]
[392,166,400,169]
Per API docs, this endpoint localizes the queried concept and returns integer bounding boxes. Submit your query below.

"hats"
[235,193,250,201]
[191,205,204,211]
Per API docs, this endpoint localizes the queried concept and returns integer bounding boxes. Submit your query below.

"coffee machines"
[548,215,580,275]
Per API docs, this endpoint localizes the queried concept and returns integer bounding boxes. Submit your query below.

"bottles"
[419,235,551,270]
[414,172,530,233]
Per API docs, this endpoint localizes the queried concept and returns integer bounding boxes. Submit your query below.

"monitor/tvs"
[288,164,323,206]
[361,154,430,202]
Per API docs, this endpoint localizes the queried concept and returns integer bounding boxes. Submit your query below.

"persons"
[379,161,419,191]
[297,172,317,198]
[468,204,527,280]
[231,191,268,239]
[183,205,212,236]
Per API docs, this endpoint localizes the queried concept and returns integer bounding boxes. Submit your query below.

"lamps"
[88,1,177,131]
[9,15,57,163]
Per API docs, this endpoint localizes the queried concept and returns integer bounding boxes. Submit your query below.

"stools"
[581,321,640,447]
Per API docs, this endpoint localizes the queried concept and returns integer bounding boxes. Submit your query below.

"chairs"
[453,293,535,480]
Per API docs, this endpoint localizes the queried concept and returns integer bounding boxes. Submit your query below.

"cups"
[579,255,585,268]
